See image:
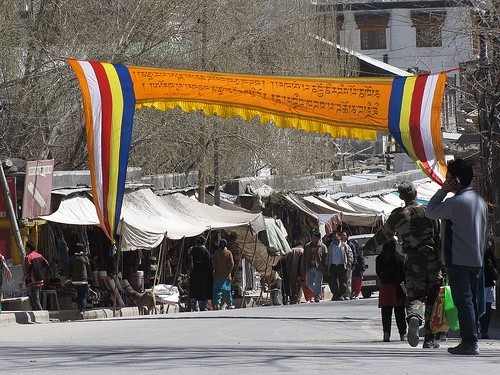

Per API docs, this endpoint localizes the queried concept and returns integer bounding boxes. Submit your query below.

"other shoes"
[483,333,490,339]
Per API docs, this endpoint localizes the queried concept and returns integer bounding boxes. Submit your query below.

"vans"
[345,233,394,298]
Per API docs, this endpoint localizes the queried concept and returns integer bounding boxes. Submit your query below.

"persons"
[347,239,365,301]
[0,254,13,289]
[339,229,358,302]
[187,235,215,310]
[19,239,50,311]
[478,246,498,340]
[373,238,409,343]
[358,180,441,349]
[67,242,92,312]
[325,231,353,302]
[423,158,490,355]
[286,241,307,304]
[301,231,327,303]
[212,239,237,311]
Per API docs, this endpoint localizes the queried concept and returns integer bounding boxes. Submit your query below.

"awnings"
[38,187,293,320]
[253,178,457,239]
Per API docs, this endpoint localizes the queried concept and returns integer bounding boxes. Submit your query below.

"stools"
[40,290,60,310]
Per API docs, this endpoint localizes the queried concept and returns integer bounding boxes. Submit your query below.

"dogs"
[127,291,159,315]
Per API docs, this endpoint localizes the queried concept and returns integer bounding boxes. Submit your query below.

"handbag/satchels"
[445,275,460,330]
[399,281,408,300]
[430,278,449,335]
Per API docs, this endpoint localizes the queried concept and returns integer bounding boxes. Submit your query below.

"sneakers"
[408,317,419,347]
[447,341,480,355]
[383,332,391,341]
[424,340,441,348]
[401,335,408,341]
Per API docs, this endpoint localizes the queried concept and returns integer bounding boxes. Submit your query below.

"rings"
[445,181,448,184]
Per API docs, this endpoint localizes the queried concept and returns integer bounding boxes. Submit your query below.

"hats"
[397,181,416,195]
